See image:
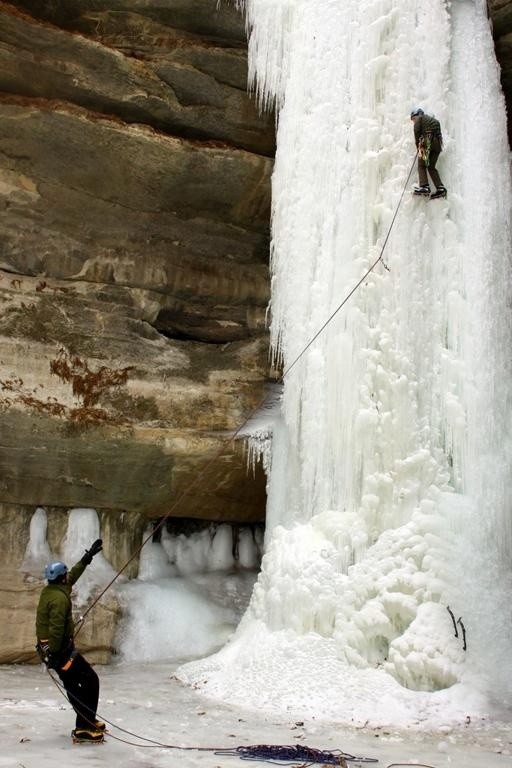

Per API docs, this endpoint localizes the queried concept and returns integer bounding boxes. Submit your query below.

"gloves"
[81,538,103,564]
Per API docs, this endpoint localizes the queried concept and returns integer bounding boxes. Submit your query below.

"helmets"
[411,108,424,120]
[44,560,69,580]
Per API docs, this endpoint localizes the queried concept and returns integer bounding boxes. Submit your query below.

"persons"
[411,108,447,198]
[34,539,106,741]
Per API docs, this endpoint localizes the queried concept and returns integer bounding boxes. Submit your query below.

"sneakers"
[90,720,106,729]
[431,185,446,198]
[74,728,104,742]
[414,185,431,194]
